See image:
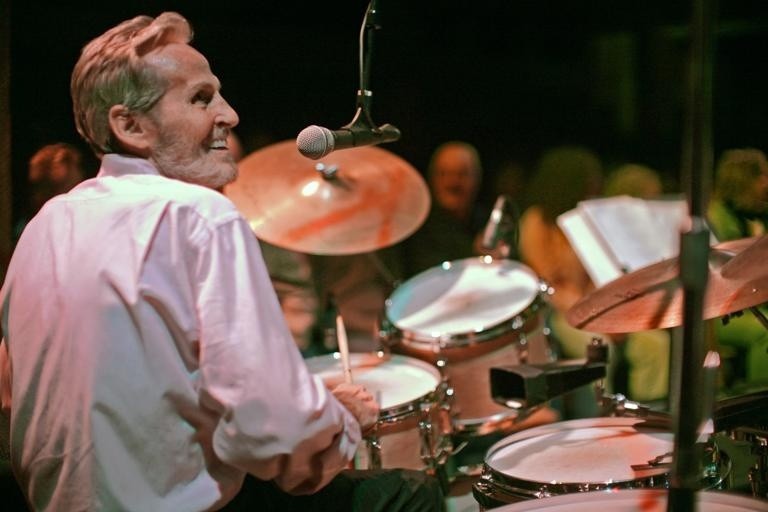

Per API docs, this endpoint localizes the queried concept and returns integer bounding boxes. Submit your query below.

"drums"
[377,258,559,436]
[300,352,457,472]
[471,416,732,507]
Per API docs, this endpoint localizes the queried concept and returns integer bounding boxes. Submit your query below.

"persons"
[22,131,768,410]
[0,8,448,510]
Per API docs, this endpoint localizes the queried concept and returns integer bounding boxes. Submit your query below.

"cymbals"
[220,140,435,257]
[566,238,768,332]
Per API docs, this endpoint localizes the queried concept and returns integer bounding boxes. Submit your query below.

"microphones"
[297,124,400,160]
[482,193,507,249]
